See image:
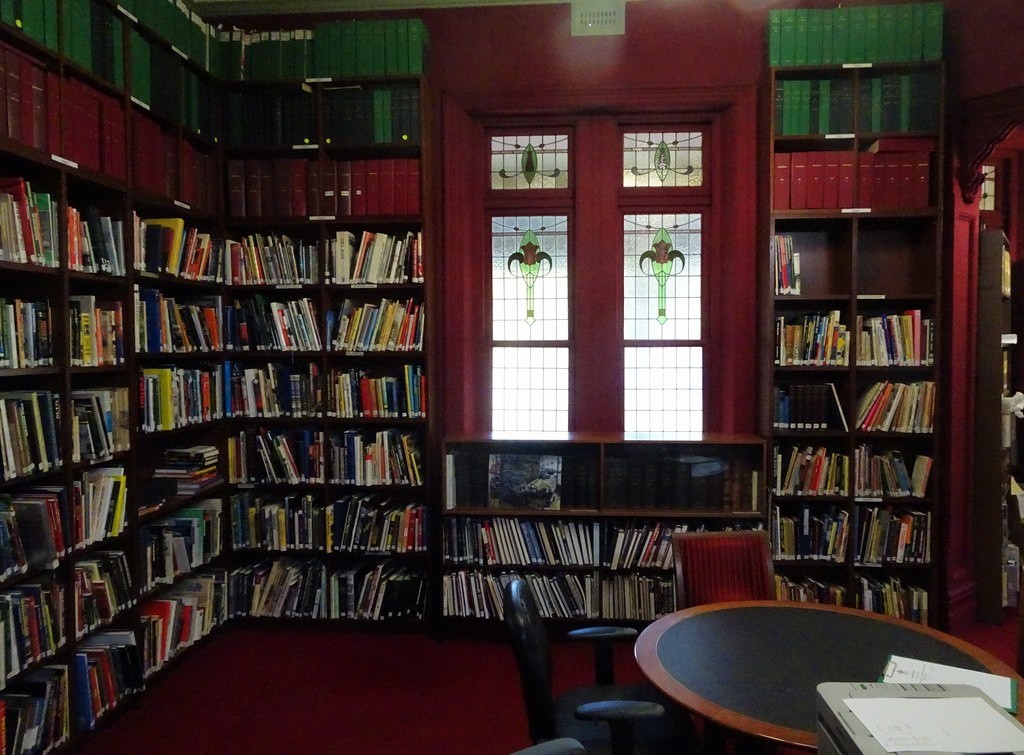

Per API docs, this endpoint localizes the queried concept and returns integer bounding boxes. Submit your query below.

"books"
[0,174,1024,755]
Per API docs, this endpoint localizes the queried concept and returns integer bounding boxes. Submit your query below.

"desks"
[633,600,1024,755]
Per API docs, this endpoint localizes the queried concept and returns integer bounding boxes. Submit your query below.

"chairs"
[502,580,702,755]
[671,531,777,755]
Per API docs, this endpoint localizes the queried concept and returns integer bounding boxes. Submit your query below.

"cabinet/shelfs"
[973,229,1022,623]
[440,433,767,638]
[0,0,445,755]
[759,58,946,634]
